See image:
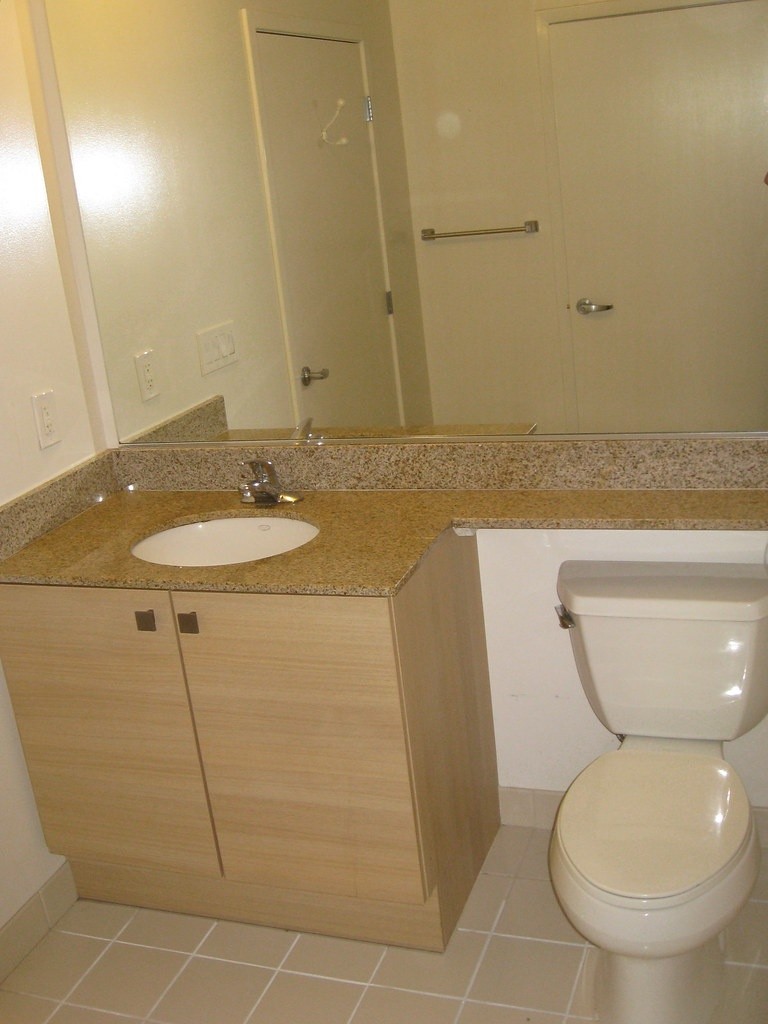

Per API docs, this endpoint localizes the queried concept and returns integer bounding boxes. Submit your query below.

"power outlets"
[32,389,63,451]
[134,349,163,403]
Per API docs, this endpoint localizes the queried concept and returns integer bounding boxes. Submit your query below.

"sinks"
[129,510,320,567]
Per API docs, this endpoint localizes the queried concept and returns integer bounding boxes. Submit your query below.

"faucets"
[237,459,282,504]
[290,417,326,446]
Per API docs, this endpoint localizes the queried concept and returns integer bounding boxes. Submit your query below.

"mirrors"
[43,0,768,447]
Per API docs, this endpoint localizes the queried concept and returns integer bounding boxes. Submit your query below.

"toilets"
[548,559,768,1023]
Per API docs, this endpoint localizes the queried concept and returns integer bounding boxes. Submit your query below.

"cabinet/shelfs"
[0,522,501,956]
